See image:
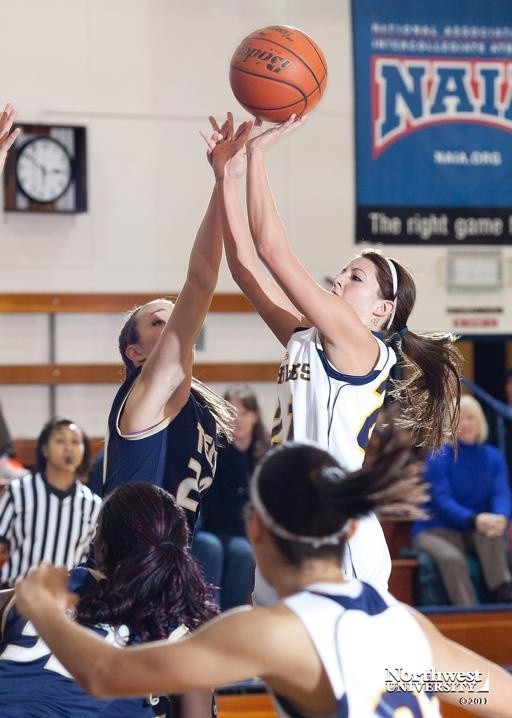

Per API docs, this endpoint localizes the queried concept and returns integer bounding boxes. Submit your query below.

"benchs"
[8,433,512,717]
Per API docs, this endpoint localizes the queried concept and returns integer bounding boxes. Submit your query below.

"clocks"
[3,122,86,215]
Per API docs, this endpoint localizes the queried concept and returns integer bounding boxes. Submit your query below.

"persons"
[0,416,109,589]
[87,111,262,560]
[194,110,467,589]
[12,442,511,718]
[0,483,221,717]
[190,383,271,610]
[0,101,21,178]
[406,391,511,609]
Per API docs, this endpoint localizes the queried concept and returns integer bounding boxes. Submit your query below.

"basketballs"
[229,26,328,124]
